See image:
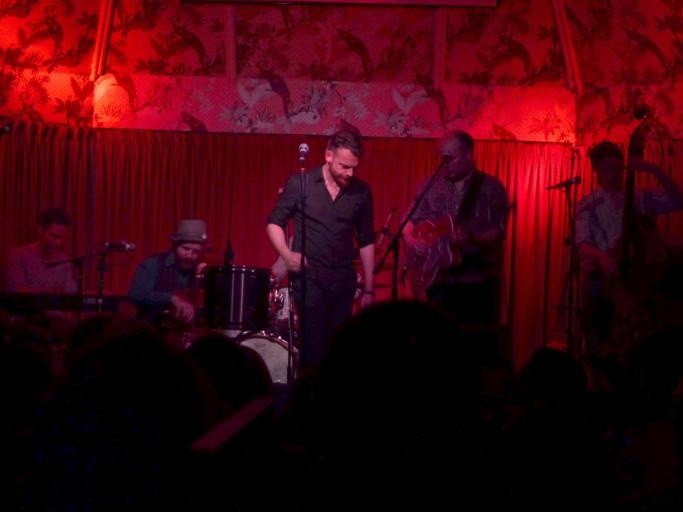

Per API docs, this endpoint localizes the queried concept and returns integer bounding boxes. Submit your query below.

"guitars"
[404,195,511,293]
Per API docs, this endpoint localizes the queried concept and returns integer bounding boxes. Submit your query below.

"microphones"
[545,176,581,190]
[299,143,309,164]
[440,152,457,161]
[105,242,136,251]
[379,208,395,243]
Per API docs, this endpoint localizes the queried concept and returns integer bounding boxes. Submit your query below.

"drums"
[270,285,300,341]
[231,330,303,385]
[200,263,272,333]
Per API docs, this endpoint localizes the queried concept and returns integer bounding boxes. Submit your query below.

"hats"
[168,219,208,243]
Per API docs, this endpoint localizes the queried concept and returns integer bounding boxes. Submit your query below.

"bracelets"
[364,290,375,296]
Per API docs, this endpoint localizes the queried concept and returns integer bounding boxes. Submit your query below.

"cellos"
[599,126,669,363]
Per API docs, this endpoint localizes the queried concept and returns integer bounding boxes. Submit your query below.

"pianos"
[0,293,129,313]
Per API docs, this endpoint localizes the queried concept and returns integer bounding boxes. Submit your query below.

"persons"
[0,298,683,511]
[126,218,211,349]
[5,207,80,341]
[400,128,510,324]
[271,237,295,279]
[265,130,377,370]
[571,140,682,392]
